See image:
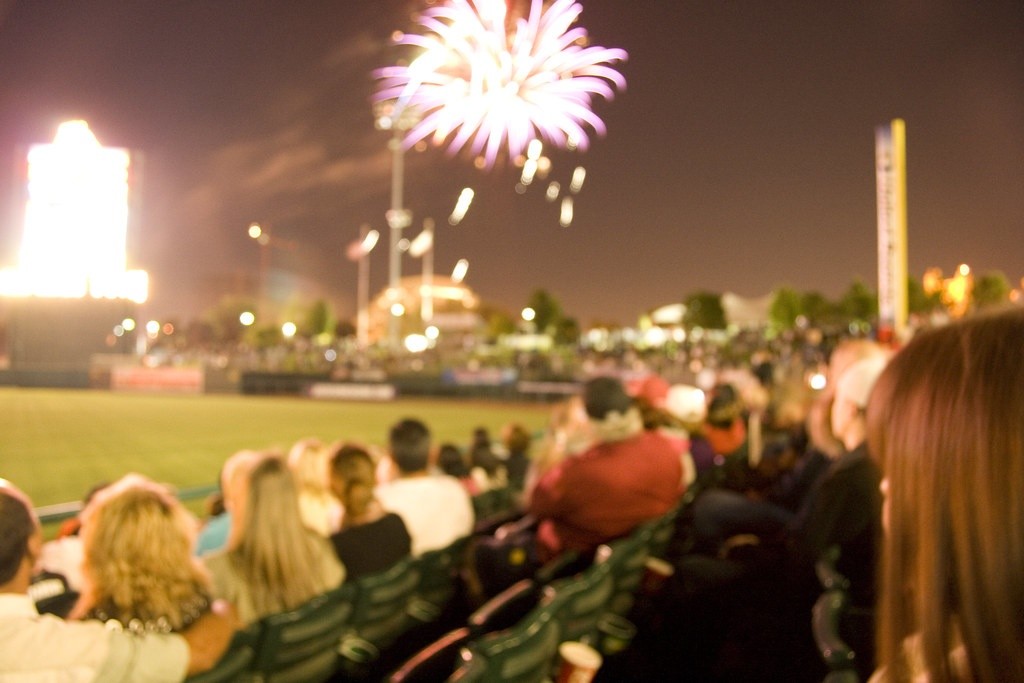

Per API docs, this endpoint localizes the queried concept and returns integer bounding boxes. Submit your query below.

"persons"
[0,335,884,683]
[872,307,1024,683]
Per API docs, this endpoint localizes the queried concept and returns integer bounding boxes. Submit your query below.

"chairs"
[26,444,856,683]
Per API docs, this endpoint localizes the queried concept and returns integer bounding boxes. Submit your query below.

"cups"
[554,641,602,682]
[642,556,674,597]
